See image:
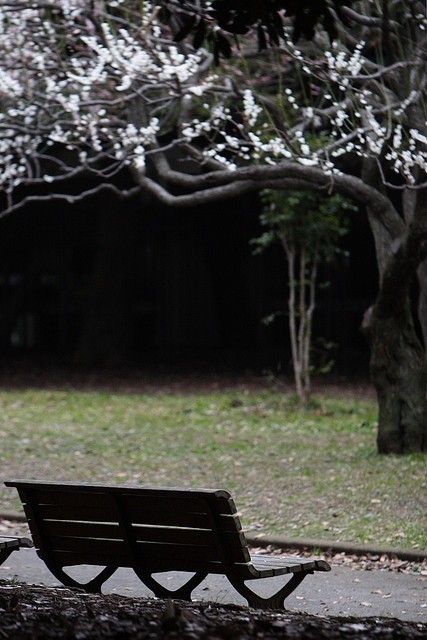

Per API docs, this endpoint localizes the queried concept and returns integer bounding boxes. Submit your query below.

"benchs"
[1,536,33,566]
[6,481,330,617]
